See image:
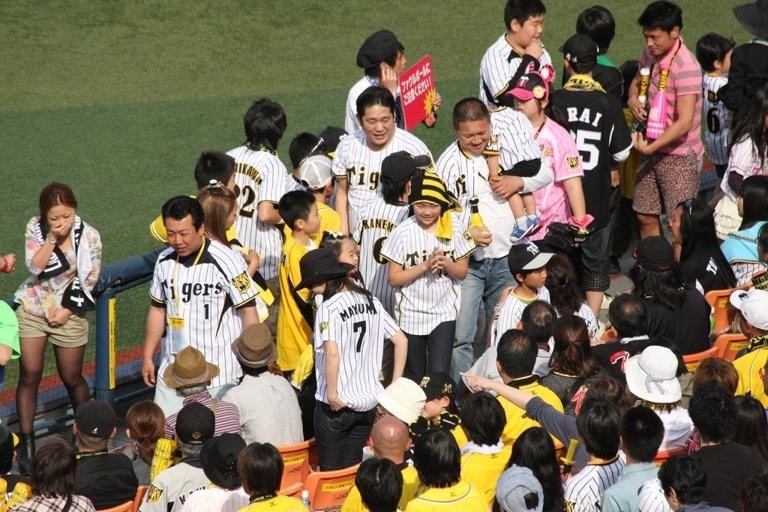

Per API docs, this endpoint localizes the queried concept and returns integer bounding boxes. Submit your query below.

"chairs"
[89,280,766,511]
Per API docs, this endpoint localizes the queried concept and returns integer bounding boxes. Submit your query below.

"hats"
[507,241,558,277]
[297,152,334,192]
[174,400,249,493]
[73,399,118,438]
[623,344,683,404]
[733,0,768,42]
[318,124,350,153]
[504,72,551,101]
[379,150,432,184]
[229,323,278,369]
[376,376,427,425]
[556,31,604,56]
[418,371,460,403]
[356,27,398,69]
[291,247,354,294]
[714,43,768,113]
[162,343,220,391]
[409,167,450,209]
[633,234,676,272]
[730,288,768,330]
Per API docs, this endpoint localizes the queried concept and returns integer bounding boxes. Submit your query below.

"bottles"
[299,491,311,509]
[468,196,485,228]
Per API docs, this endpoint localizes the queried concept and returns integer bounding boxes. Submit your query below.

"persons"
[0,1,768,512]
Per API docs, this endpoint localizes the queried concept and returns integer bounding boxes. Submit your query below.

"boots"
[13,430,42,477]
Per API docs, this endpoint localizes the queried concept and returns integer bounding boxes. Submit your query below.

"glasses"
[309,134,325,157]
[683,197,696,217]
